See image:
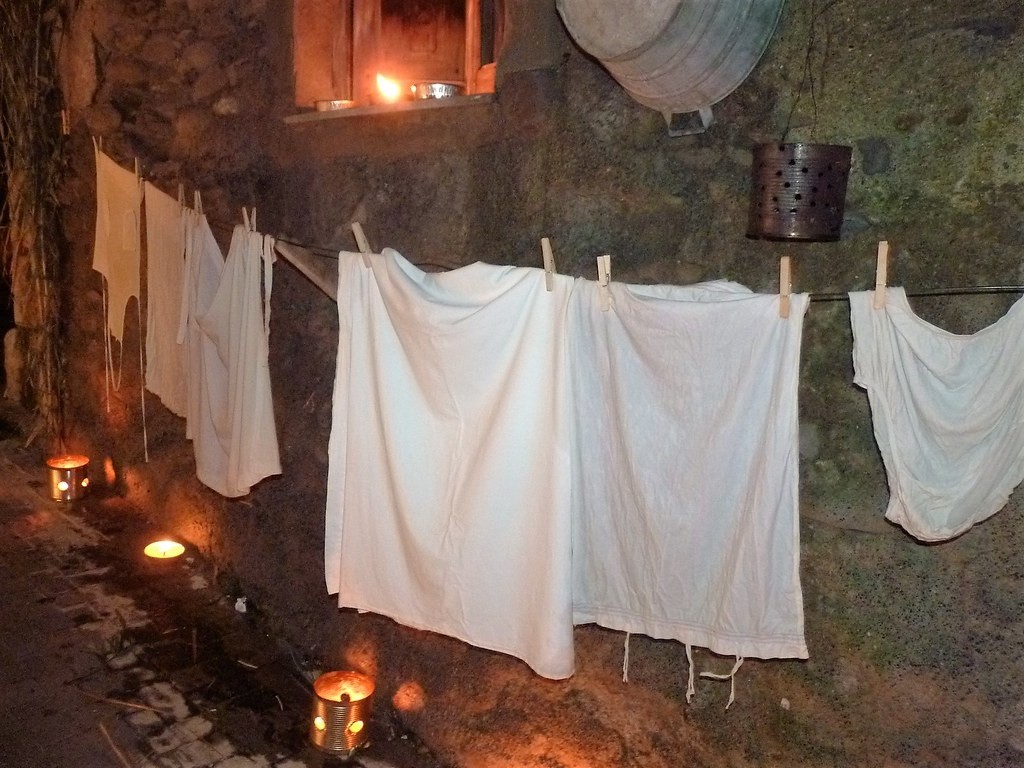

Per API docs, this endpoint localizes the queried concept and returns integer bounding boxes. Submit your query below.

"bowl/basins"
[410,83,458,100]
[315,100,353,112]
[145,541,185,564]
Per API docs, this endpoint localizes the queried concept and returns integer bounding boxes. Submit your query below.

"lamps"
[144,539,186,574]
[309,670,376,755]
[46,453,91,501]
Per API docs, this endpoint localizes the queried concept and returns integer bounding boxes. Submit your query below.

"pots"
[556,0,784,137]
